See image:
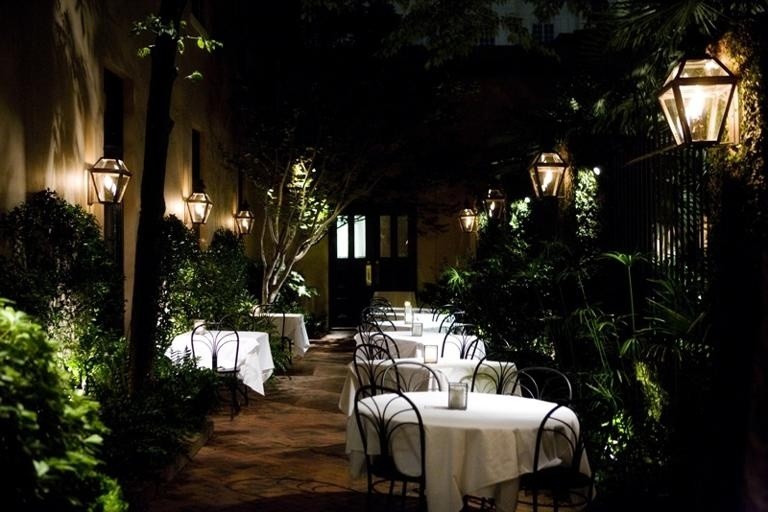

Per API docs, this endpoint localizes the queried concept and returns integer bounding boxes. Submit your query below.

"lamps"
[530,151,566,197]
[482,187,506,219]
[658,54,742,146]
[91,156,130,207]
[186,192,212,223]
[236,209,256,235]
[458,208,476,233]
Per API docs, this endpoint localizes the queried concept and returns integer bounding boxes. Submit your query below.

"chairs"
[253,304,292,362]
[419,299,440,313]
[355,384,426,512]
[362,307,388,328]
[517,398,613,512]
[503,368,572,411]
[438,311,468,333]
[371,304,396,322]
[359,321,389,359]
[191,323,248,419]
[353,344,400,397]
[432,303,462,321]
[441,323,481,358]
[368,316,396,330]
[448,317,475,334]
[382,364,442,396]
[464,337,503,359]
[369,296,393,313]
[215,312,257,331]
[369,334,401,360]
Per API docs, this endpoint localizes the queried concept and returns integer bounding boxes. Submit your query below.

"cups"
[449,383,468,410]
[194,318,205,335]
[412,323,424,337]
[252,304,260,316]
[424,345,438,364]
[404,312,415,325]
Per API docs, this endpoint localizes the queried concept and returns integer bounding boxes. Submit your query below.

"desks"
[472,350,545,398]
